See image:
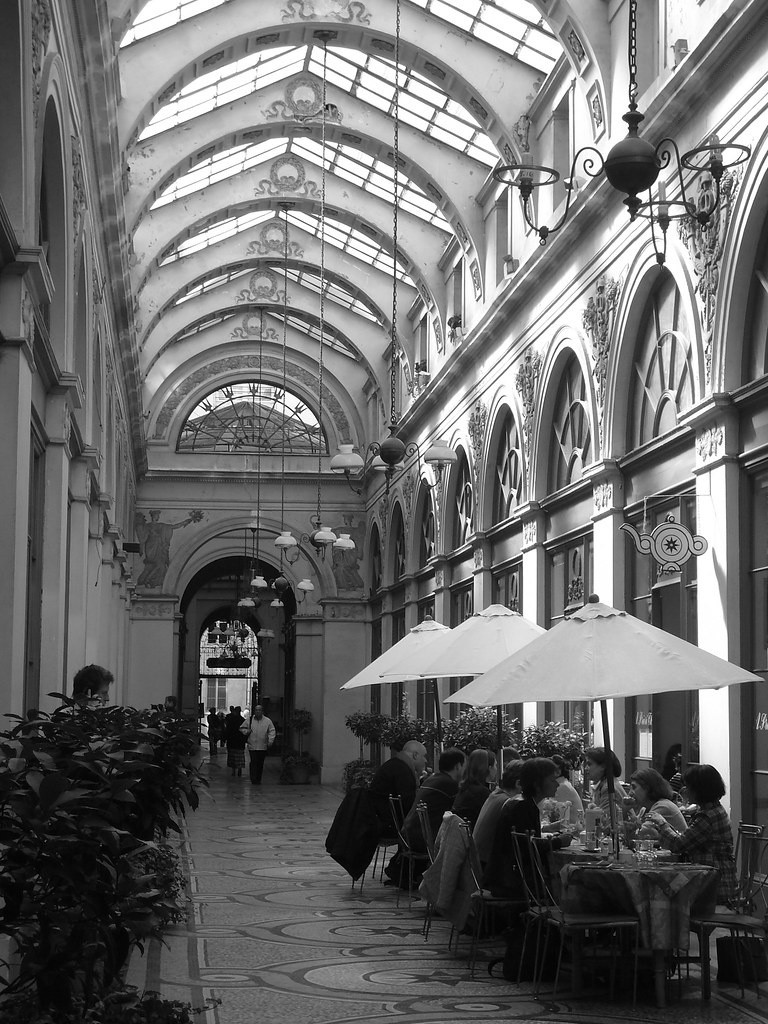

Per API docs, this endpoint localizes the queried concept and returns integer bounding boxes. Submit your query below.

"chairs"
[324,787,401,895]
[418,811,522,955]
[456,816,529,979]
[511,826,639,1007]
[684,820,766,981]
[386,795,432,912]
[673,836,768,1007]
[415,799,493,942]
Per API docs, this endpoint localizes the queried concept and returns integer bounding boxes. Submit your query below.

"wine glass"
[631,839,659,870]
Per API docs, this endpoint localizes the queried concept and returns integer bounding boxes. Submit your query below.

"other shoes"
[238,770,243,777]
[231,772,236,776]
[504,964,537,982]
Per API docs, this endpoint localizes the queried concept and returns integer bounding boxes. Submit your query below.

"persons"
[622,767,689,833]
[217,706,248,776]
[207,707,220,751]
[369,740,433,878]
[164,695,177,711]
[401,747,468,890]
[669,752,682,789]
[451,749,497,834]
[644,765,741,906]
[238,705,276,785]
[491,757,573,983]
[472,759,525,872]
[584,747,628,807]
[73,664,115,707]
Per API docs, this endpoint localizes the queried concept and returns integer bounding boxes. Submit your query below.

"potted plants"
[278,707,321,784]
[342,709,387,793]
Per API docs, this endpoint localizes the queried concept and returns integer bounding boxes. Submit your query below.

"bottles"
[575,809,585,838]
[600,841,609,856]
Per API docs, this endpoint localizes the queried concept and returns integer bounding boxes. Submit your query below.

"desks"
[540,820,719,1009]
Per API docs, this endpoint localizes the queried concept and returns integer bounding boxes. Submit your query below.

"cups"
[586,832,596,851]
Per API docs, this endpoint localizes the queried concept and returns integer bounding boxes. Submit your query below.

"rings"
[648,814,651,818]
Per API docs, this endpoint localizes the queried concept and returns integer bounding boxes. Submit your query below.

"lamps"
[272,30,356,566]
[492,0,751,269]
[250,203,315,604]
[330,0,458,496]
[209,591,275,660]
[237,305,284,616]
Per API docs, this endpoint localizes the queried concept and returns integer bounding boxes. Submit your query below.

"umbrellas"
[339,615,453,754]
[379,604,548,785]
[443,593,766,851]
